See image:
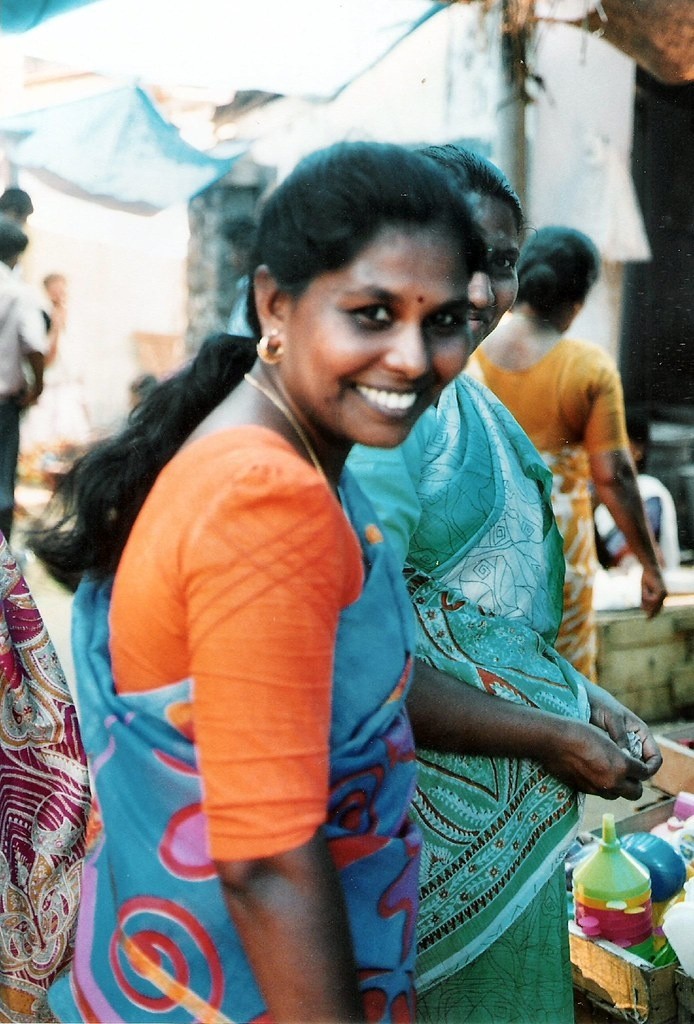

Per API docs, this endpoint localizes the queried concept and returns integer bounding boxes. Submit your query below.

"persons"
[0,138,681,1024]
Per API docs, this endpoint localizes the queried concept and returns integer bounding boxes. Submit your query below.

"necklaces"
[243,372,325,481]
